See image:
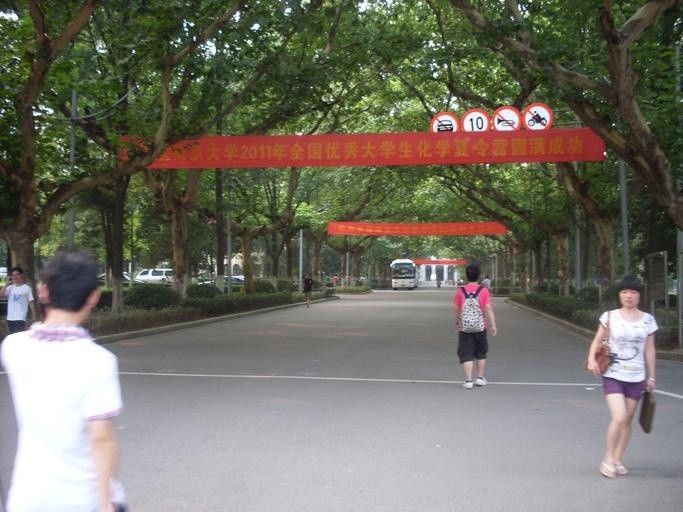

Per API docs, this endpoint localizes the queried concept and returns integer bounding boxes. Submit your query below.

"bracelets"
[647,377,657,382]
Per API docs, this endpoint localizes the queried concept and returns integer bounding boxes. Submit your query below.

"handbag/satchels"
[584,346,611,374]
[639,391,657,434]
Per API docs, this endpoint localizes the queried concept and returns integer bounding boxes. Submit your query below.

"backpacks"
[459,286,485,333]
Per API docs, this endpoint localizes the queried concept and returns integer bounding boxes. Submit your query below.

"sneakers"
[476,376,489,386]
[463,380,474,389]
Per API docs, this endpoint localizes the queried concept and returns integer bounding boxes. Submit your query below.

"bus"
[388,258,419,291]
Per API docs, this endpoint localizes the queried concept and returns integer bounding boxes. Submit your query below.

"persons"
[584,274,657,478]
[480,274,491,292]
[302,272,313,308]
[0,267,37,334]
[0,242,129,512]
[453,263,497,389]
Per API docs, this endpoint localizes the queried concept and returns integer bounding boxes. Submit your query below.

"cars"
[0,254,248,287]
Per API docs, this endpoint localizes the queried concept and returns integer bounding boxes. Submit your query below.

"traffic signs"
[461,108,490,131]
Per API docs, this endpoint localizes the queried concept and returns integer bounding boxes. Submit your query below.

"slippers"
[601,460,628,480]
